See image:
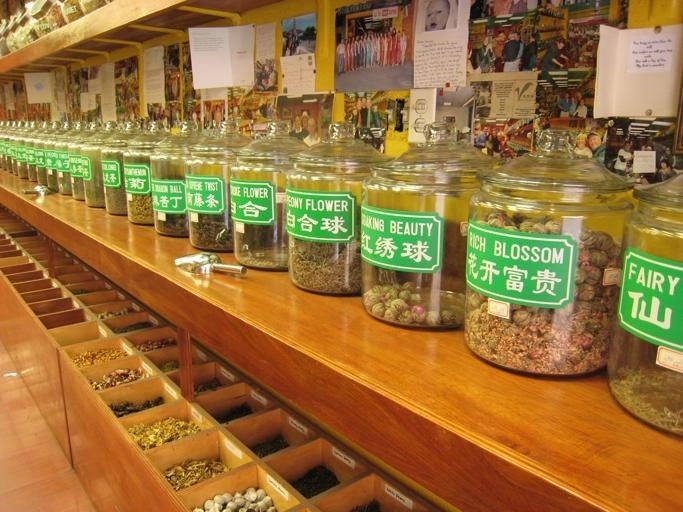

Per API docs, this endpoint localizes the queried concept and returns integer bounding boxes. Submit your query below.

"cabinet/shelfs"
[0,1,683,511]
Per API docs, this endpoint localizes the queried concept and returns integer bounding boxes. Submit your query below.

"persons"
[114,0,678,185]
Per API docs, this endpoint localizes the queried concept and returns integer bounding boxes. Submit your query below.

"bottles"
[359,119,499,335]
[1,117,253,252]
[0,0,108,56]
[605,169,683,440]
[462,126,634,380]
[228,120,314,273]
[285,118,395,299]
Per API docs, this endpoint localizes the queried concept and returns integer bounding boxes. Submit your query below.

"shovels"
[174,251,247,275]
[23,184,58,194]
